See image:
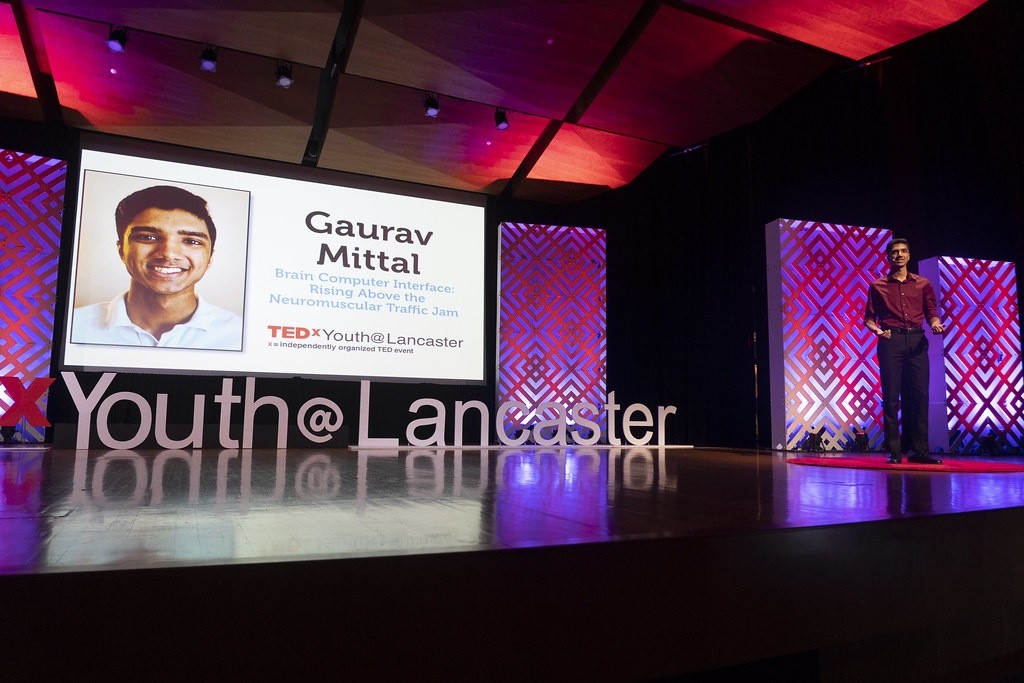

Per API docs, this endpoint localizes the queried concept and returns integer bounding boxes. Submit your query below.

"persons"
[70,185,242,351]
[864,239,946,465]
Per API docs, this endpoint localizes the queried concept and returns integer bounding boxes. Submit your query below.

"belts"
[881,327,925,334]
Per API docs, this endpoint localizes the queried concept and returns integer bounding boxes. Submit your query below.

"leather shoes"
[887,456,900,464]
[907,457,941,464]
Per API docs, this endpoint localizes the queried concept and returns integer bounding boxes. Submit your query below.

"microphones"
[888,256,895,261]
